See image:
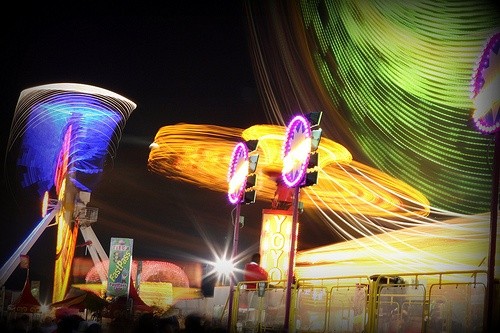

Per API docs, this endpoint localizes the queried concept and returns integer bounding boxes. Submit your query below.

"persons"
[241,253,270,290]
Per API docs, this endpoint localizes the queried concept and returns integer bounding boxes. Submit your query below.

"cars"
[359,274,406,297]
